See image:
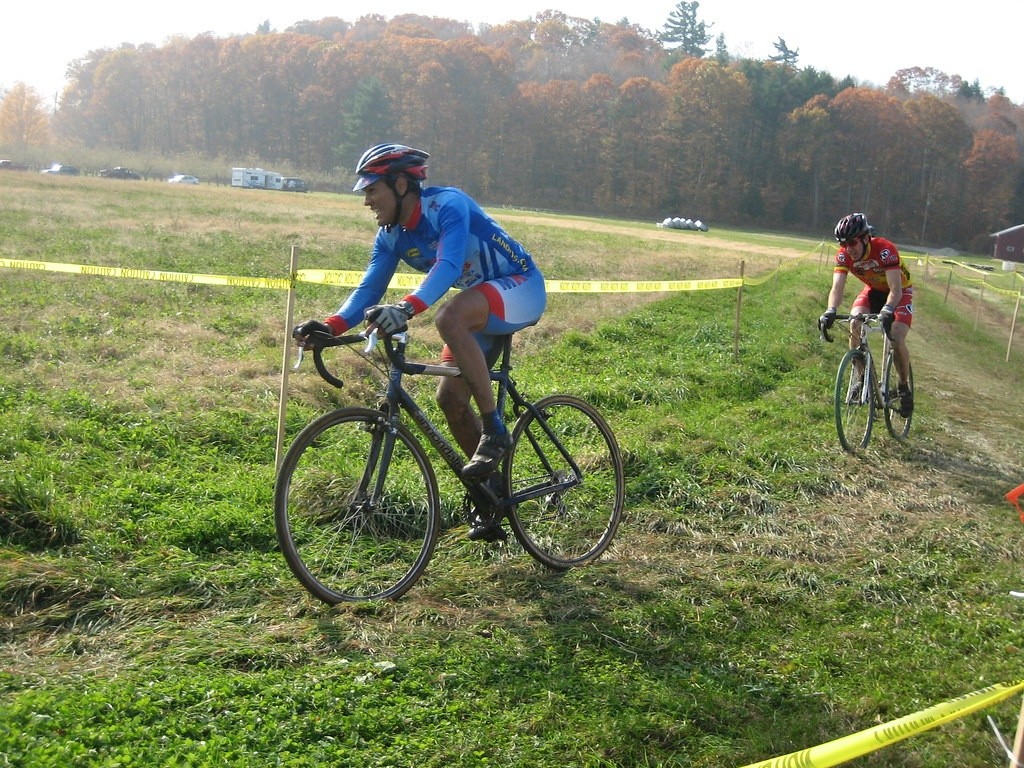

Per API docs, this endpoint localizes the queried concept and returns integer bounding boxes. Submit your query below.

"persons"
[292,144,547,539]
[818,213,915,418]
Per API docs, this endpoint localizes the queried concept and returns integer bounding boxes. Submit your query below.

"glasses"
[840,236,863,248]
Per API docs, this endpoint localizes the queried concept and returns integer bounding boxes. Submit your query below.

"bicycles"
[272,310,626,609]
[816,311,915,459]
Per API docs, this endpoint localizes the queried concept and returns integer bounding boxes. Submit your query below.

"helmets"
[357,143,431,181]
[834,212,868,243]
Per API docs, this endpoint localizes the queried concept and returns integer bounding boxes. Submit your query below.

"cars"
[42,165,81,177]
[100,166,141,180]
[167,175,200,184]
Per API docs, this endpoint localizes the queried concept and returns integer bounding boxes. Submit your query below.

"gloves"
[817,307,837,330]
[878,305,895,332]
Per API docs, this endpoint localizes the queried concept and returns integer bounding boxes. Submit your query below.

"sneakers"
[851,376,868,399]
[896,381,913,419]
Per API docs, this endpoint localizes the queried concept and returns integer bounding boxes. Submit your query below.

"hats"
[353,172,383,192]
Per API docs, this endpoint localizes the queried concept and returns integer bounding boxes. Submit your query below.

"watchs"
[395,301,414,320]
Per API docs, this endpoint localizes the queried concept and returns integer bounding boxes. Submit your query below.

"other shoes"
[461,423,514,479]
[467,500,520,541]
